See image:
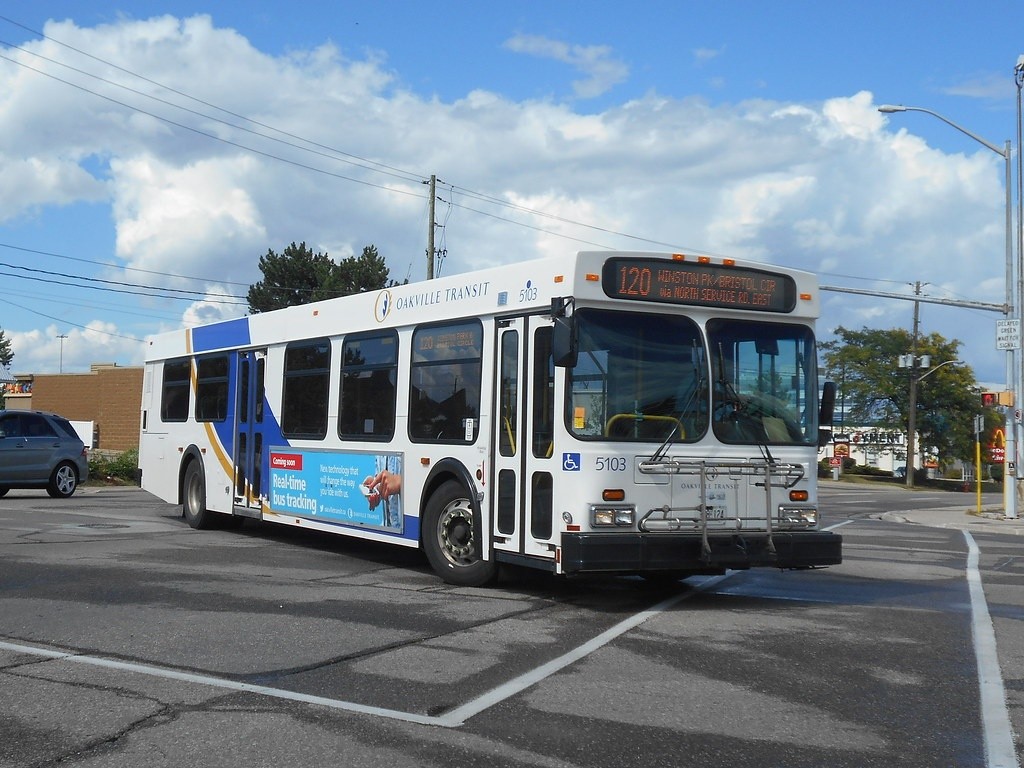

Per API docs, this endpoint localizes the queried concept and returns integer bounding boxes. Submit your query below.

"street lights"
[56,333,68,373]
[879,98,1016,520]
[906,359,965,486]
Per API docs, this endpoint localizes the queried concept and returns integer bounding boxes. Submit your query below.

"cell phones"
[359,485,380,496]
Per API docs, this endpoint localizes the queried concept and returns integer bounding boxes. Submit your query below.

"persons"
[362,455,403,528]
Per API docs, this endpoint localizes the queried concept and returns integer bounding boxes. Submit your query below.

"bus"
[138,248,848,590]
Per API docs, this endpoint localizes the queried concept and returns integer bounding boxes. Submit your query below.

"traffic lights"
[981,392,997,406]
[998,391,1015,408]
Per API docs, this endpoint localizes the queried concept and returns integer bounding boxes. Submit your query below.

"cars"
[0,409,88,499]
